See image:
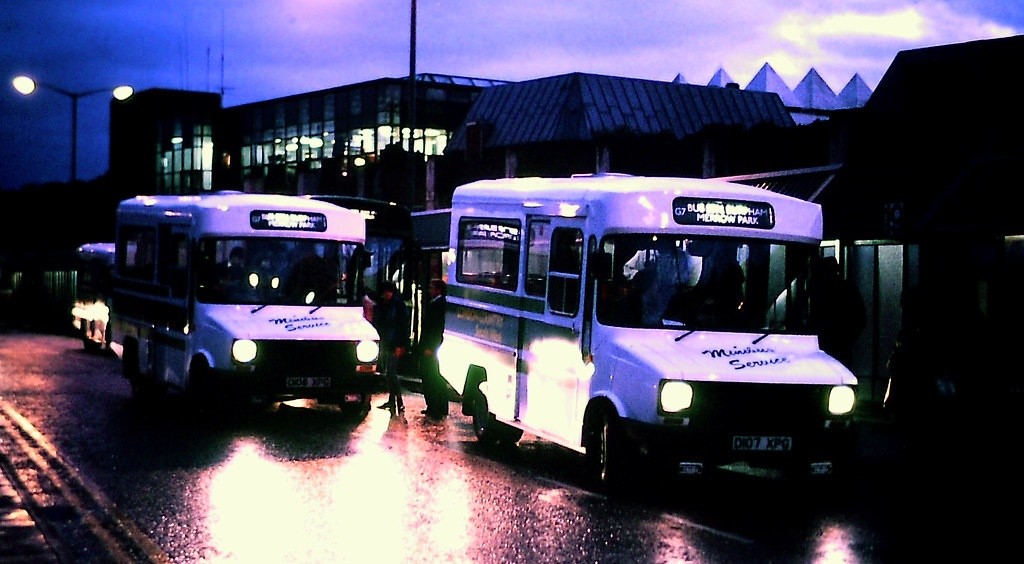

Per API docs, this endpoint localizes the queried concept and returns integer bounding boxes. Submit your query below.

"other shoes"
[421,405,448,424]
[377,401,405,411]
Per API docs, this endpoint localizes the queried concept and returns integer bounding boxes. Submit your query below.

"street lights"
[12,75,134,177]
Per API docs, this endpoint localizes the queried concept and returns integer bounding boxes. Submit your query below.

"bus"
[71,243,134,354]
[437,171,860,491]
[111,190,381,418]
[302,197,412,375]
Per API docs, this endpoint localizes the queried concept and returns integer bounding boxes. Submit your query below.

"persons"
[418,277,449,417]
[374,281,411,411]
